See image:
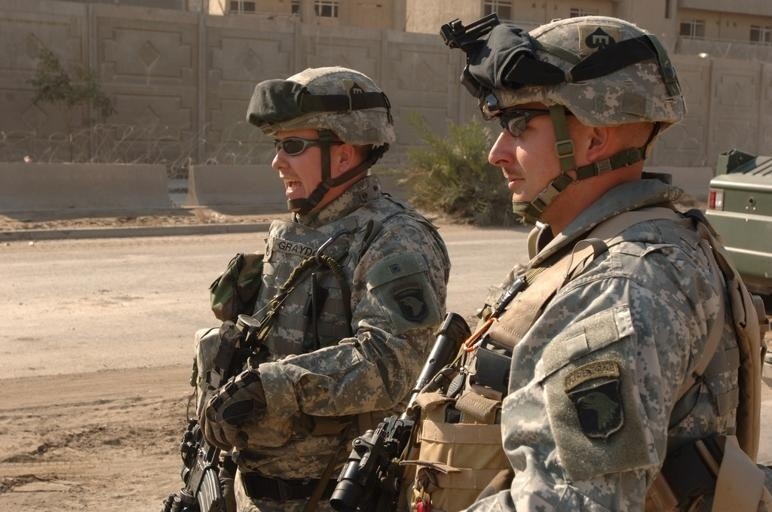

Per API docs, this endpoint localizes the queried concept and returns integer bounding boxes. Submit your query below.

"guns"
[331,312,471,512]
[161,313,260,512]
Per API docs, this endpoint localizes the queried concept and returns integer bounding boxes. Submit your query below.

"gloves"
[200,367,266,451]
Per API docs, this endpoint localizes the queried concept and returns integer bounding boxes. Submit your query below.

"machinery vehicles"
[706,148,772,320]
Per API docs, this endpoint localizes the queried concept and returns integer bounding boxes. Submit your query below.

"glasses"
[499,107,574,138]
[274,136,344,156]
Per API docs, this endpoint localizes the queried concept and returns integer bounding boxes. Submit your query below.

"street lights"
[696,49,715,164]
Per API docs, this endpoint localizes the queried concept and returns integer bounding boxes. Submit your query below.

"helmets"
[262,67,396,147]
[481,14,688,135]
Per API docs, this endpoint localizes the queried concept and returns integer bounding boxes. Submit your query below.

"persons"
[202,66,451,512]
[401,14,763,512]
